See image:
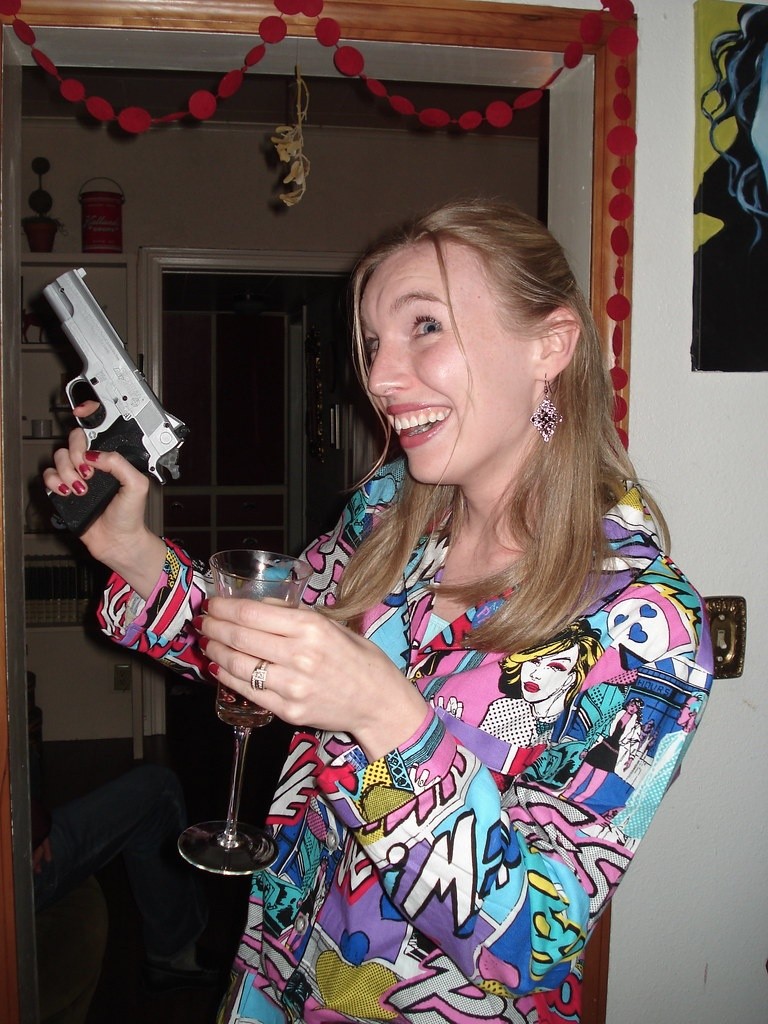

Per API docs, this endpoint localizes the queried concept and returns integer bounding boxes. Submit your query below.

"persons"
[41,200,713,1024]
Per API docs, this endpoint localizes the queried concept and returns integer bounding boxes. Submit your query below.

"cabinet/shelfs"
[18,254,144,759]
[162,309,290,557]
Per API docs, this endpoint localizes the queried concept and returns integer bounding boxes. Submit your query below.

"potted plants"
[20,156,66,253]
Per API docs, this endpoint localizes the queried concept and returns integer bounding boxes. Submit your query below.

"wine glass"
[177,550,314,875]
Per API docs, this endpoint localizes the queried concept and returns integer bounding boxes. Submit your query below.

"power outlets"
[114,663,132,691]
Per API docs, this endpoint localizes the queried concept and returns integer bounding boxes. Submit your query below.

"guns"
[39,262,195,544]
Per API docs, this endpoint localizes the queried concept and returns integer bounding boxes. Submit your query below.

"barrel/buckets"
[78,176,125,252]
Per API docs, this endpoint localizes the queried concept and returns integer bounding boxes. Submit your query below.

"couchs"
[36,876,108,1024]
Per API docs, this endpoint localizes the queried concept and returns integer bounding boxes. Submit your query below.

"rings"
[251,661,271,690]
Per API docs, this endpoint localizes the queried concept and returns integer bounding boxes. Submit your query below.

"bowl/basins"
[32,419,52,437]
